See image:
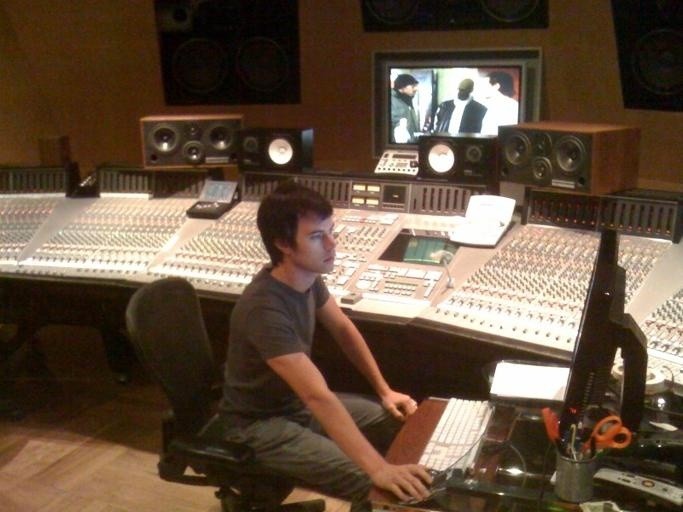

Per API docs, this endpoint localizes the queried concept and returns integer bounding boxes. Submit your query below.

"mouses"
[426,467,443,484]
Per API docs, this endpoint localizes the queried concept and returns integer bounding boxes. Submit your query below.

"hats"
[394,74,419,90]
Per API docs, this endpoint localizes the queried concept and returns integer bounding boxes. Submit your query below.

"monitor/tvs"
[375,51,531,151]
[557,225,683,482]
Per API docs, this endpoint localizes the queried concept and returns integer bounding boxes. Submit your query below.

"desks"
[368,399,639,512]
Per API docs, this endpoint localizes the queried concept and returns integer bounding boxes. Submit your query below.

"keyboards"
[417,396,496,477]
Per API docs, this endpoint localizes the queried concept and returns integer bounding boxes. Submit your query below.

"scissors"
[580,416,632,454]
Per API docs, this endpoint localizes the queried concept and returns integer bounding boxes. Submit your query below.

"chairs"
[125,276,325,512]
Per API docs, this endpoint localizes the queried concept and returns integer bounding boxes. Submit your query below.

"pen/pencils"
[541,406,584,460]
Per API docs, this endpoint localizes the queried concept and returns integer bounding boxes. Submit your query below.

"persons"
[218,182,434,512]
[430,78,488,134]
[390,74,419,144]
[481,72,519,135]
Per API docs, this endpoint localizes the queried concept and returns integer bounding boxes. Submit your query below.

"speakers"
[236,125,314,173]
[139,113,243,172]
[418,132,499,185]
[498,120,642,197]
